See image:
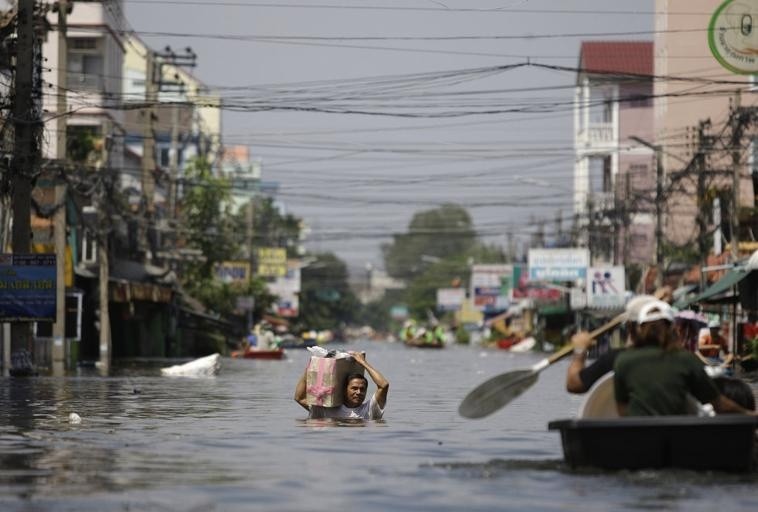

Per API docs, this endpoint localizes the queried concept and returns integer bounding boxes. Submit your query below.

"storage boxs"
[305,351,366,407]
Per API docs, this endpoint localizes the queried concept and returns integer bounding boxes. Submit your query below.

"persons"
[293,351,391,423]
[245,323,285,352]
[612,302,758,419]
[406,318,448,347]
[566,283,670,394]
[674,310,758,374]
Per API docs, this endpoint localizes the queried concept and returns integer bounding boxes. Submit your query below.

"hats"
[622,294,720,329]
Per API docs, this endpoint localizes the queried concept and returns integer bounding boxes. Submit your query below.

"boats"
[402,337,444,351]
[229,339,321,359]
[548,413,758,474]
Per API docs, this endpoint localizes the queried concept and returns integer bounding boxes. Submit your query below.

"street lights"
[419,251,474,300]
[625,132,711,311]
[510,172,623,262]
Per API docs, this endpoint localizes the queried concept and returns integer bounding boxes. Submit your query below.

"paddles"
[458,288,670,419]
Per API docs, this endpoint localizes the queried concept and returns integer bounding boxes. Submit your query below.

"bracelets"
[571,347,589,356]
